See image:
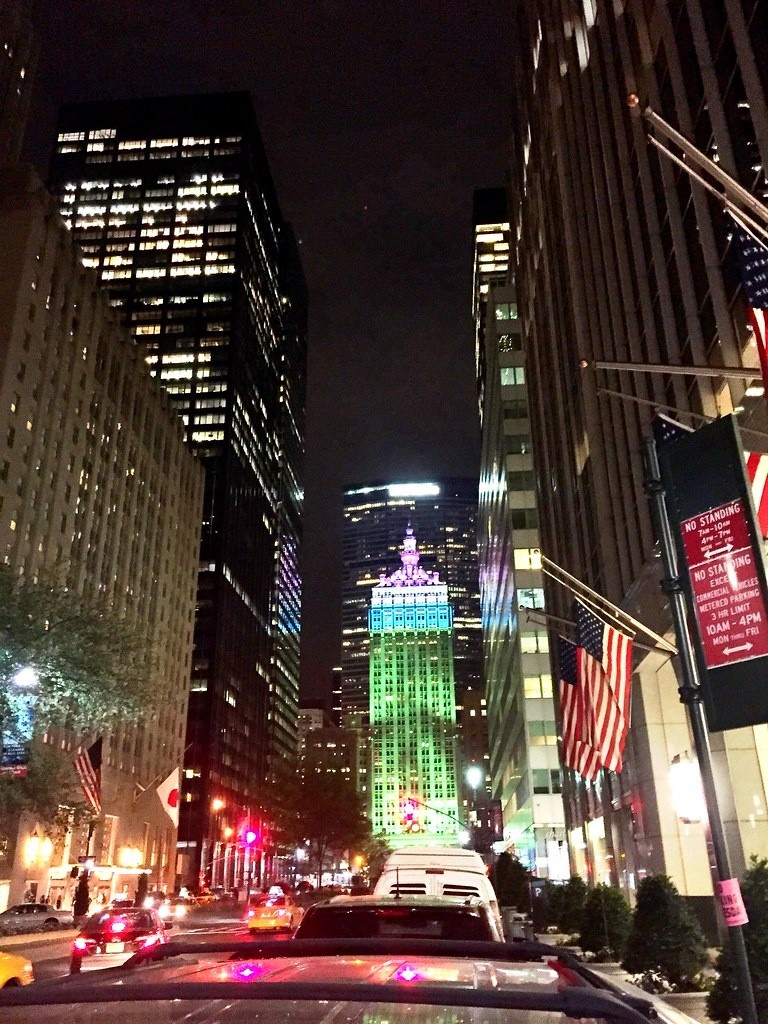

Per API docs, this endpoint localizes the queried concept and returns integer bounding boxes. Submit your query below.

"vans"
[369,848,505,938]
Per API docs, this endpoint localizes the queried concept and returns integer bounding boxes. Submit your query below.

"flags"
[727,210,768,388]
[75,737,102,812]
[557,636,601,782]
[574,598,632,773]
[156,767,180,827]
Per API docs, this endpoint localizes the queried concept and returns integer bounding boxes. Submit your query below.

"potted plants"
[536,873,712,1024]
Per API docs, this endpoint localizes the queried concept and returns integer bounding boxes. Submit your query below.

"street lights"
[244,826,258,904]
[406,797,478,852]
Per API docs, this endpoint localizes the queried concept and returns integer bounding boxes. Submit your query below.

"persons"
[40,895,62,910]
[102,894,106,904]
[233,888,238,900]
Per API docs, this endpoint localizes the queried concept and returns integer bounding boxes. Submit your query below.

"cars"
[296,881,314,895]
[70,908,171,974]
[245,894,305,932]
[221,886,235,898]
[0,947,35,989]
[103,899,135,908]
[251,887,262,895]
[158,896,201,928]
[0,902,73,932]
[196,891,216,904]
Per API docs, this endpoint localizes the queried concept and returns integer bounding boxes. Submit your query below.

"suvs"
[0,937,700,1023]
[292,865,504,942]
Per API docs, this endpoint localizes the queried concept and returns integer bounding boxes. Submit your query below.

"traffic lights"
[405,804,413,821]
[410,821,419,834]
